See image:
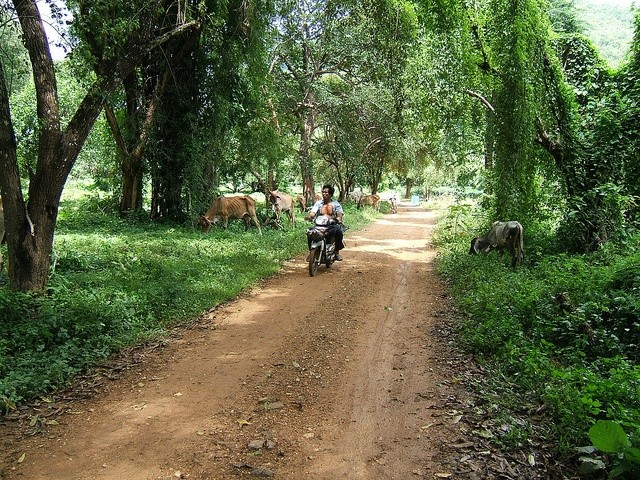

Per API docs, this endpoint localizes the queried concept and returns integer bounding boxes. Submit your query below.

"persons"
[303,183,346,263]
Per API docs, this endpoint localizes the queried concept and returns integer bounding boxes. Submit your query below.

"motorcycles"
[306,215,349,276]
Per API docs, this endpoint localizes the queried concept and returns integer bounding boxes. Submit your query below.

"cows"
[468,221,526,269]
[357,195,380,211]
[197,194,262,235]
[389,195,400,213]
[268,188,295,224]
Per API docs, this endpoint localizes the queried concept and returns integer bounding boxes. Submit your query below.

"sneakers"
[335,254,343,261]
[306,255,310,262]
